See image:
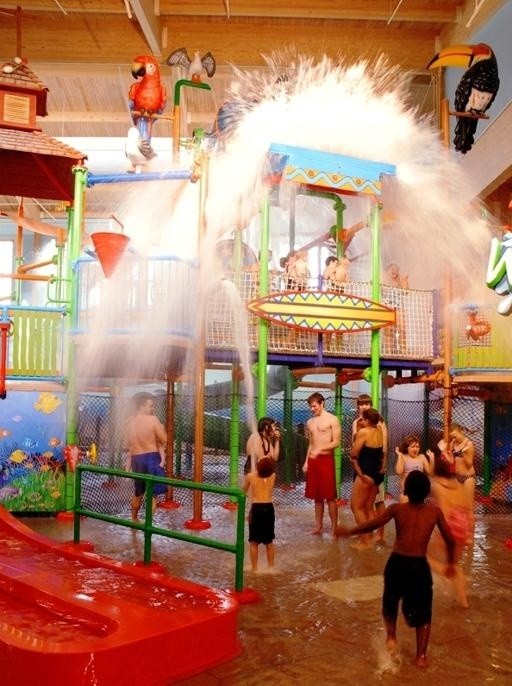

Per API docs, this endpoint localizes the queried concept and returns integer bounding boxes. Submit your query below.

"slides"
[130,365,351,467]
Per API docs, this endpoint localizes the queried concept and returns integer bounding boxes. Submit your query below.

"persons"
[283,249,312,349]
[124,396,168,521]
[249,248,278,345]
[298,392,474,664]
[125,399,166,471]
[329,258,350,292]
[379,263,411,354]
[243,455,277,571]
[244,417,281,476]
[285,251,310,289]
[322,256,344,353]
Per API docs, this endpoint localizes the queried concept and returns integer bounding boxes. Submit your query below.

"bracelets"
[274,437,280,440]
